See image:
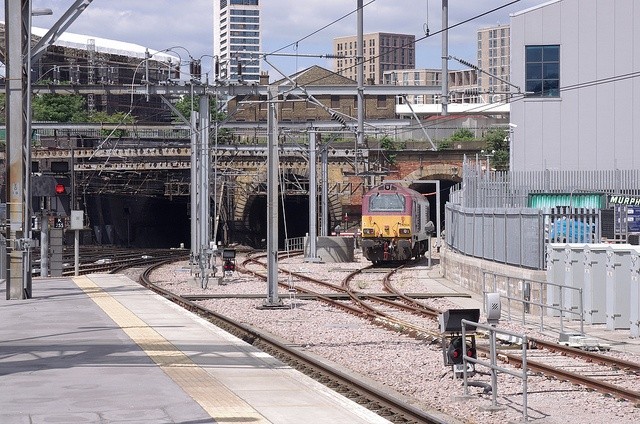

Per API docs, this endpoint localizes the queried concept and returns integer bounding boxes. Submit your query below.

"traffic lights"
[55,183,66,194]
[222,262,234,270]
[447,338,476,363]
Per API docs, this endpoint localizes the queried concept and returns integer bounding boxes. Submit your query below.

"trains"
[360,182,431,266]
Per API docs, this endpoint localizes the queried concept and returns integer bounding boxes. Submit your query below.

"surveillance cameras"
[504,137,507,142]
